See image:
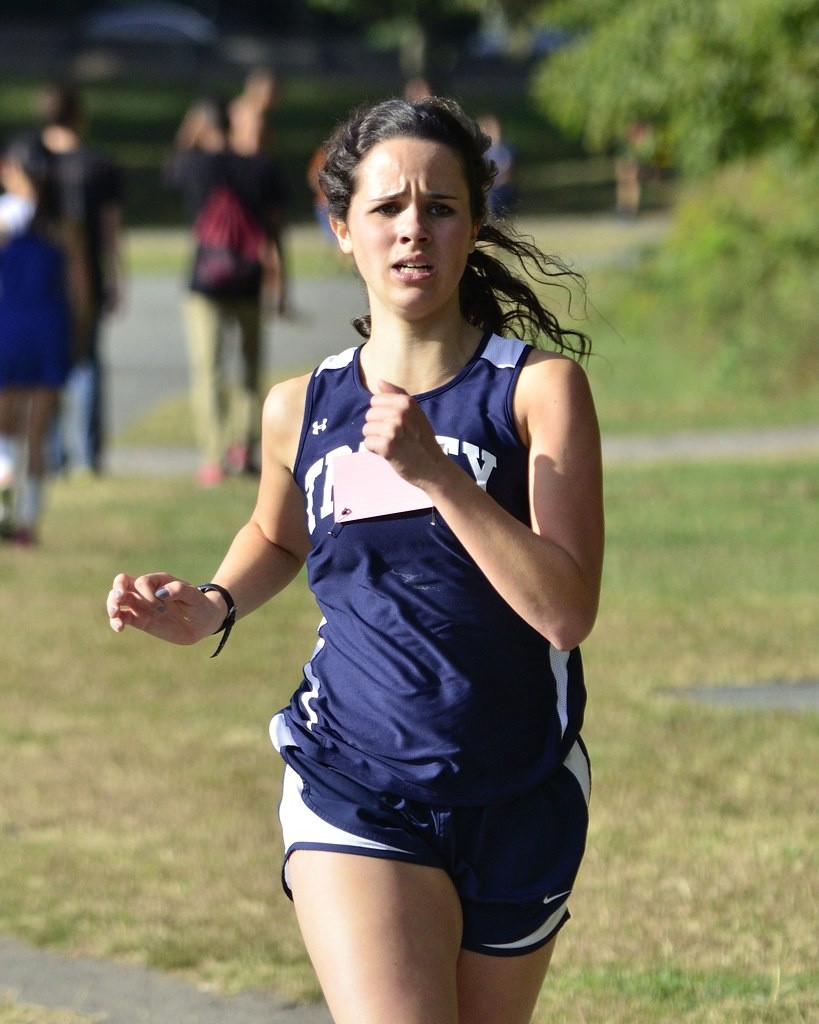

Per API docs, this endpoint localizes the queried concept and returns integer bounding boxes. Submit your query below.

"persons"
[0,66,669,549]
[104,96,607,1024]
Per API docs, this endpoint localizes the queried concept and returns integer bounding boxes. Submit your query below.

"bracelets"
[197,583,235,657]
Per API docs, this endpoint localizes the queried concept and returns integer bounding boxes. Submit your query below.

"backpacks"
[190,156,263,301]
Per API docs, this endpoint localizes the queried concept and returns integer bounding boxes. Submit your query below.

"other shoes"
[226,445,251,475]
[194,463,225,484]
